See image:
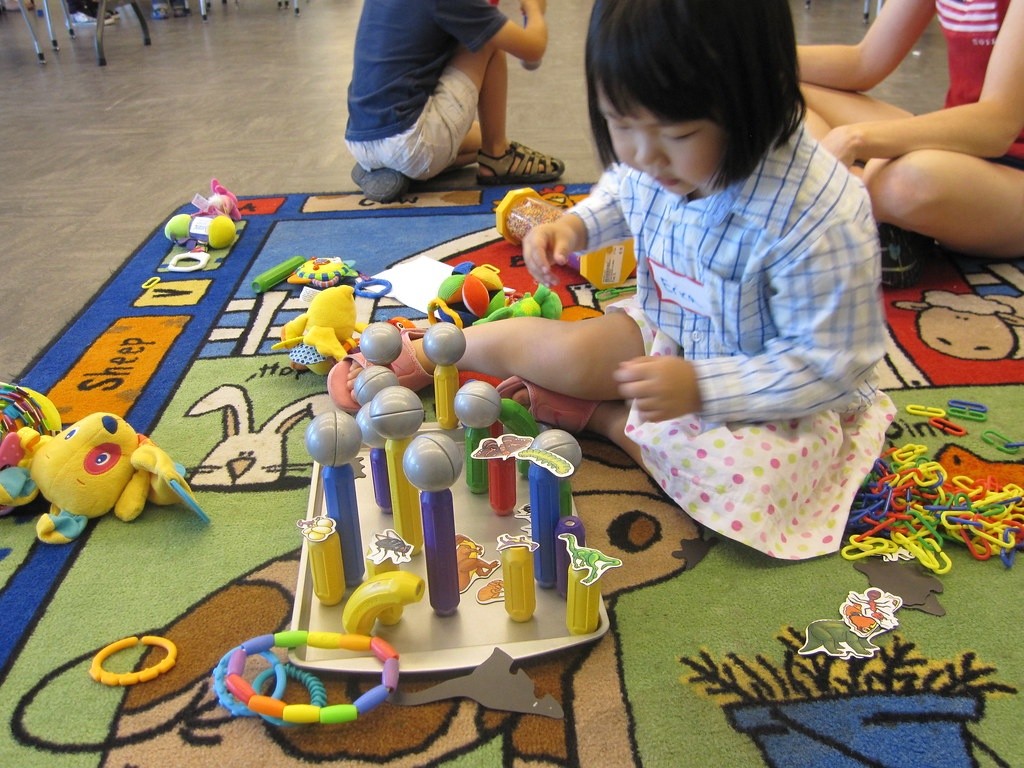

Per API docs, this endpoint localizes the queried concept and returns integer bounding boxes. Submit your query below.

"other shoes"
[103,7,121,24]
[151,3,169,19]
[875,222,924,286]
[64,11,99,28]
[174,8,192,17]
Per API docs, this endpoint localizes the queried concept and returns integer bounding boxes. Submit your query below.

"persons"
[63,0,186,26]
[346,0,566,201]
[325,0,897,559]
[794,0,1024,287]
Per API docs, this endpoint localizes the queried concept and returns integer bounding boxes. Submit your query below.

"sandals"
[350,161,411,203]
[475,140,565,183]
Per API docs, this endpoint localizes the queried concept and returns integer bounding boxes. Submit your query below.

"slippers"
[327,322,434,413]
[494,374,603,435]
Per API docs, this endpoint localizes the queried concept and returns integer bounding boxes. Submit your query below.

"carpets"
[0,184,1024,768]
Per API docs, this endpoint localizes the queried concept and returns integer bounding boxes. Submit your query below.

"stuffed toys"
[271,284,368,375]
[0,411,210,542]
[192,179,241,219]
[471,284,563,325]
